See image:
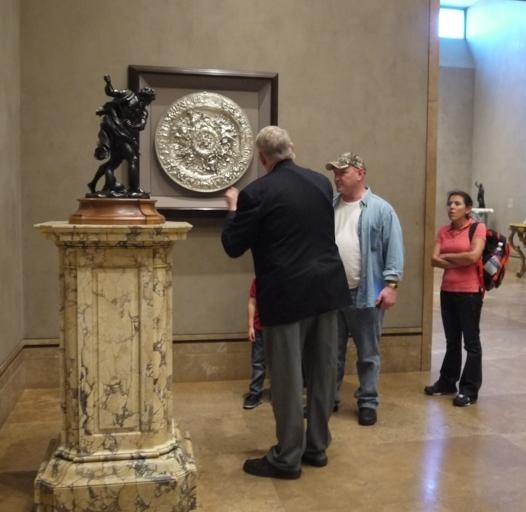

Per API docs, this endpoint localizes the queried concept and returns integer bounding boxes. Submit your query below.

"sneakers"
[244,393,263,409]
[424,381,458,395]
[454,393,476,406]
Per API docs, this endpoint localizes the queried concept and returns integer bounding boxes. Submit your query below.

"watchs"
[388,281,399,290]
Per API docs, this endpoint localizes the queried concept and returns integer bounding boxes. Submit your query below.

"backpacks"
[469,222,509,298]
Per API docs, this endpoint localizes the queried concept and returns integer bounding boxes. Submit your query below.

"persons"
[241,278,272,409]
[422,191,487,407]
[219,126,352,481]
[475,180,485,208]
[85,74,158,196]
[321,151,406,426]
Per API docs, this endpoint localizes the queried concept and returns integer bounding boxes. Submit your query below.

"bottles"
[495,242,503,261]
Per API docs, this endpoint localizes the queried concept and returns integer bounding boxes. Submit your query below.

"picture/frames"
[129,65,278,216]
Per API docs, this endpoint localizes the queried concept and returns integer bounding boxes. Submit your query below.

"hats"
[326,152,366,171]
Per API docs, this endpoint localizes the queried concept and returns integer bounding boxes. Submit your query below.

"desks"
[32,219,198,512]
[472,208,495,226]
[508,224,526,278]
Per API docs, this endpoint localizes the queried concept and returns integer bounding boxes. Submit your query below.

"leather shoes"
[244,456,301,479]
[358,407,376,424]
[304,400,339,417]
[301,450,327,466]
